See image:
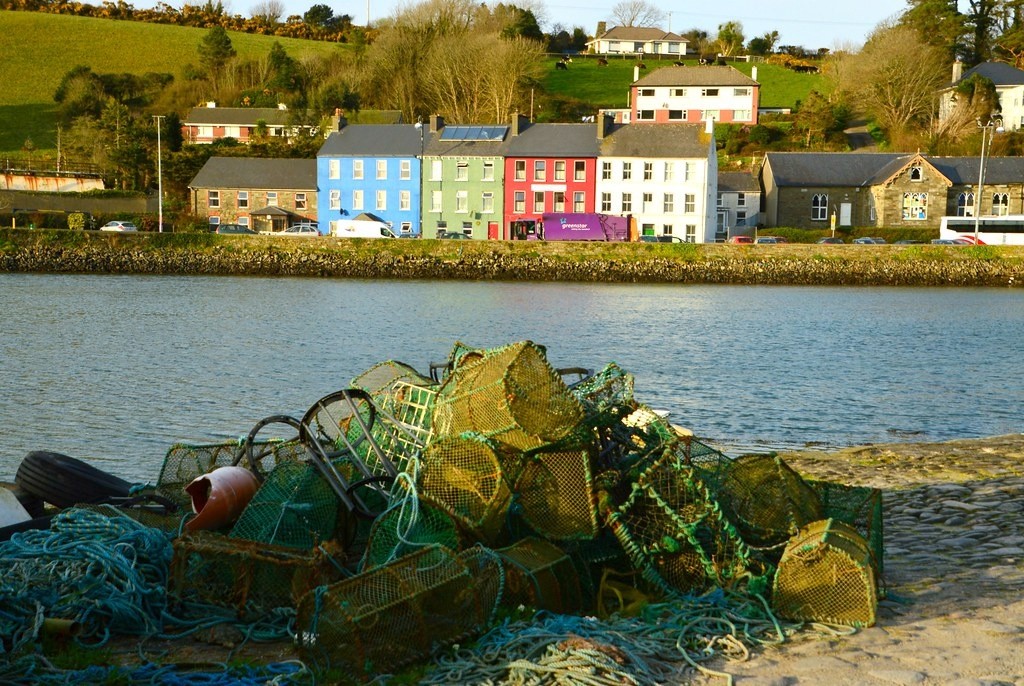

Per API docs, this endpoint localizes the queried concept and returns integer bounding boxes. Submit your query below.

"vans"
[335,218,399,239]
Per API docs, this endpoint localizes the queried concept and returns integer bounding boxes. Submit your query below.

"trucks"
[513,212,633,241]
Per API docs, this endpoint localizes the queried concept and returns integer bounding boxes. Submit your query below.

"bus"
[939,214,1024,244]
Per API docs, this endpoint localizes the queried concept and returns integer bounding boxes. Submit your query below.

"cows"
[555,58,821,75]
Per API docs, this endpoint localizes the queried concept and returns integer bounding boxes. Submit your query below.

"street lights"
[151,115,167,233]
[981,118,1005,195]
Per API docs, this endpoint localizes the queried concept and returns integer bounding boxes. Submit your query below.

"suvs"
[215,223,257,234]
[637,235,691,243]
[100,220,139,231]
[272,224,323,237]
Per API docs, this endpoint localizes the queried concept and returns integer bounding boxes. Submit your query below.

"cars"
[398,233,422,238]
[724,235,754,245]
[438,231,472,239]
[754,237,791,244]
[852,235,987,247]
[808,235,845,244]
